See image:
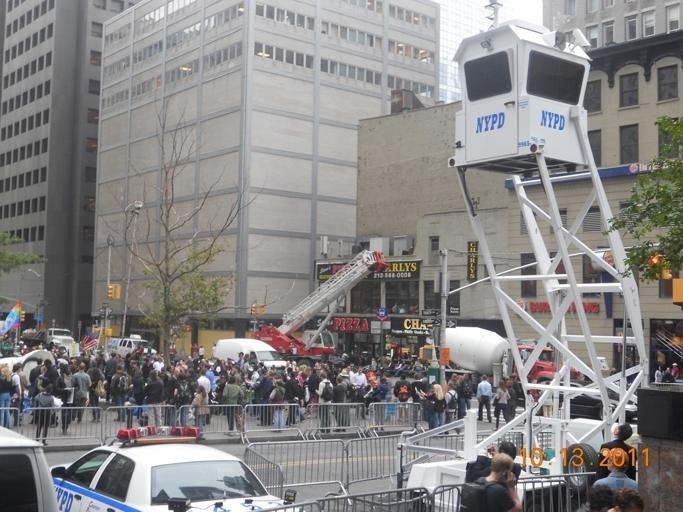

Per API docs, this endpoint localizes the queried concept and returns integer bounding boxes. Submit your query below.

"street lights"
[122,200,144,338]
[27,268,42,328]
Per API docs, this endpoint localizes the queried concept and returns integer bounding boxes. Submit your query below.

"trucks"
[20,328,74,349]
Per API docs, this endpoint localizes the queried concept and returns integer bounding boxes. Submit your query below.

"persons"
[670,362,680,381]
[654,364,664,383]
[462,441,521,484]
[660,367,675,382]
[0,337,523,437]
[587,447,638,492]
[578,481,615,512]
[600,484,645,512]
[466,452,523,512]
[593,421,637,481]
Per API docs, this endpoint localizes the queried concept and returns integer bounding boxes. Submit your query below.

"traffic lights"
[107,285,115,298]
[20,311,25,322]
[251,304,256,316]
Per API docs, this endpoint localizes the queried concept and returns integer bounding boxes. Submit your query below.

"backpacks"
[434,400,445,411]
[322,382,334,401]
[398,381,409,401]
[448,392,458,409]
[115,374,129,392]
[460,481,495,512]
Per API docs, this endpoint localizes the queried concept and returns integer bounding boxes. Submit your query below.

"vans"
[214,338,287,370]
[117,338,157,360]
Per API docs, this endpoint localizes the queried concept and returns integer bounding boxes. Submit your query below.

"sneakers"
[61,417,82,433]
[114,417,125,421]
[320,429,346,433]
[478,418,491,422]
[91,417,101,422]
[492,428,497,430]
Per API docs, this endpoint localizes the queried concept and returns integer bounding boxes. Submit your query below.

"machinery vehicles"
[255,250,390,370]
[445,327,586,402]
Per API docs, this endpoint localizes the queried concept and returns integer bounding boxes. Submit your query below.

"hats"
[602,448,629,463]
[42,359,51,366]
[448,382,454,387]
[672,363,677,365]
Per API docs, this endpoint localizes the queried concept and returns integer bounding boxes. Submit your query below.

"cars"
[560,382,638,422]
[48,424,305,512]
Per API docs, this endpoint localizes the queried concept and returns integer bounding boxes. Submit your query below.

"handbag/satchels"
[96,382,105,397]
[275,391,283,401]
[492,396,500,405]
